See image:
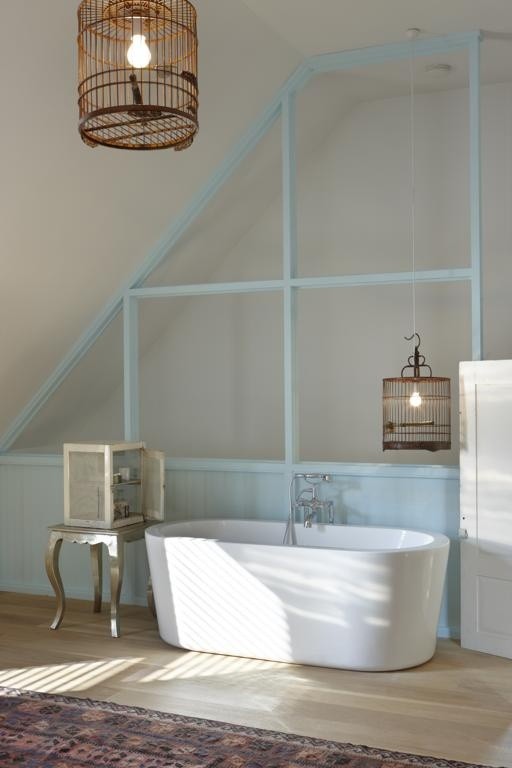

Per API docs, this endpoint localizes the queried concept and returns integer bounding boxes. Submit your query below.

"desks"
[44,520,160,638]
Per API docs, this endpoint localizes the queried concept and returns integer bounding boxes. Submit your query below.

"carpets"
[1,688,509,768]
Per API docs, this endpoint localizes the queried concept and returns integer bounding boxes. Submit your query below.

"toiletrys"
[125,504,129,517]
[113,473,121,483]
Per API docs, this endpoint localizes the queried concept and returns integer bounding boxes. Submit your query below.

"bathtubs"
[144,517,450,673]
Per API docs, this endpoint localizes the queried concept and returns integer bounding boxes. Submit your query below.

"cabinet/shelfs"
[63,440,166,528]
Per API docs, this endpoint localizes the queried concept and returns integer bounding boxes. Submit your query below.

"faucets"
[304,511,316,528]
